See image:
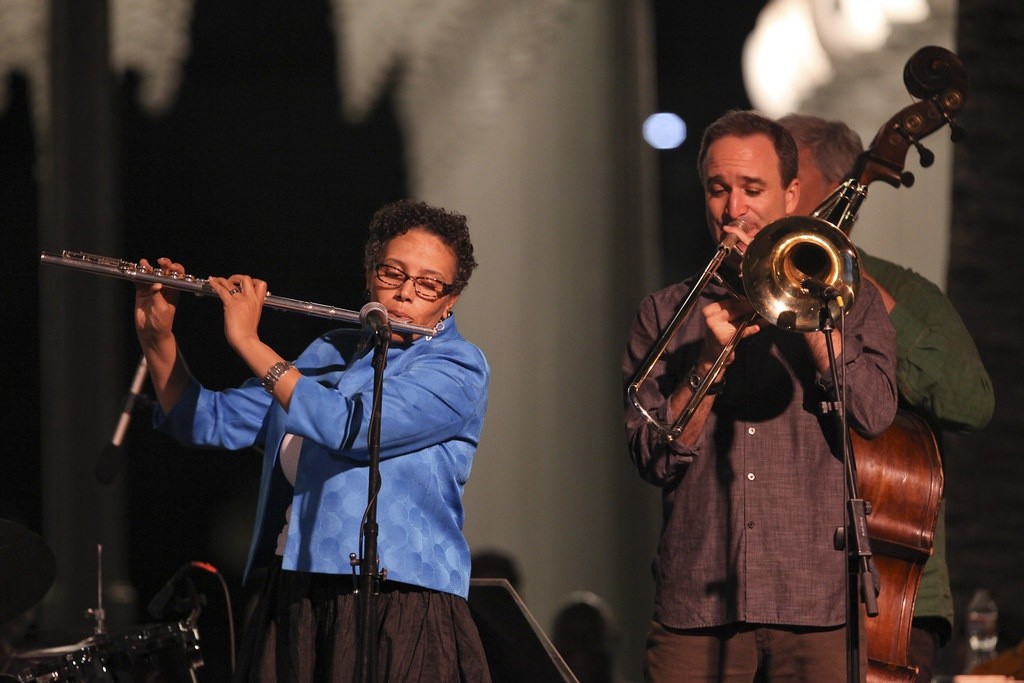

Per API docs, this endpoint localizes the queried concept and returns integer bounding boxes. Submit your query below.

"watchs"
[687,365,726,395]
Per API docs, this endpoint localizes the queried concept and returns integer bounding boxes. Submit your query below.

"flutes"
[40,250,437,339]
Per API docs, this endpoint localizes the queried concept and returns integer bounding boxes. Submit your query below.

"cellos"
[846,47,966,682]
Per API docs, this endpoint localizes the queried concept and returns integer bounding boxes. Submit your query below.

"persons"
[553,599,614,683]
[620,112,898,683]
[135,200,492,683]
[778,113,995,683]
[469,551,518,592]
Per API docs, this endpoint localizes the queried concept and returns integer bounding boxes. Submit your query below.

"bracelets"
[262,361,297,393]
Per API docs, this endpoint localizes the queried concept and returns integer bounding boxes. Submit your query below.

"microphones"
[94,355,149,486]
[149,565,190,620]
[800,277,841,301]
[359,302,392,341]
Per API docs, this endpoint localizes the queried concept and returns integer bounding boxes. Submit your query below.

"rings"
[230,288,240,295]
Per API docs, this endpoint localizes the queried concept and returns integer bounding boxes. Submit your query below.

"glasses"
[370,258,461,298]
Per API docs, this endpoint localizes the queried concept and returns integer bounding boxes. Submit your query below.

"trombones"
[627,180,870,442]
